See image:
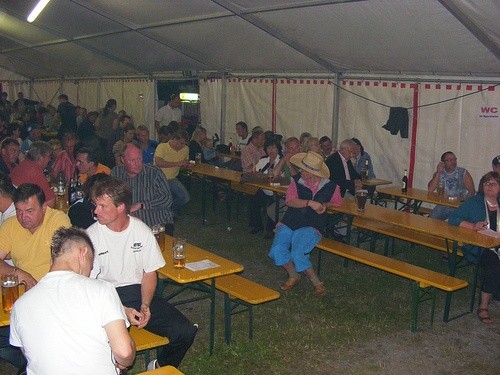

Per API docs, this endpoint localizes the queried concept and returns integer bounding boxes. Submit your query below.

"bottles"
[401,170,408,192]
[363,159,369,179]
[68,179,82,205]
[228,138,233,153]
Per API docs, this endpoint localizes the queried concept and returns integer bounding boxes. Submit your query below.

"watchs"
[140,200,144,210]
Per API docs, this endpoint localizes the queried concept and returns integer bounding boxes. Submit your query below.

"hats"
[290,151,330,177]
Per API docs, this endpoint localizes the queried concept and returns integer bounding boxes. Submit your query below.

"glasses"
[486,182,497,186]
[442,158,458,162]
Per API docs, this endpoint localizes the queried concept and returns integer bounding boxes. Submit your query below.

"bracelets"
[307,200,310,207]
[13,267,19,274]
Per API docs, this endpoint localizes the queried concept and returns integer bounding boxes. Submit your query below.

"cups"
[196,152,202,166]
[152,224,165,252]
[449,195,458,204]
[268,169,274,185]
[58,177,66,196]
[355,189,368,211]
[171,239,186,269]
[235,144,241,156]
[360,168,366,183]
[437,181,445,197]
[1,274,27,314]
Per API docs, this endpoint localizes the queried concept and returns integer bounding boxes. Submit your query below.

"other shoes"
[264,230,272,238]
[280,277,299,290]
[249,226,256,233]
[314,281,326,295]
[146,359,160,370]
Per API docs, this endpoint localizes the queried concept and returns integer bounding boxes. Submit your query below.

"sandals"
[476,307,492,324]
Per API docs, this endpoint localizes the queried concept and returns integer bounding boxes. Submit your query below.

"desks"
[157,233,245,355]
[190,163,500,322]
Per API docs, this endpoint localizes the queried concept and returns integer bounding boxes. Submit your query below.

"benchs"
[127,274,281,370]
[272,216,468,332]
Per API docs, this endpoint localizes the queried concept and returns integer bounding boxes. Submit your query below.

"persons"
[220,121,376,244]
[268,151,342,296]
[84,177,197,371]
[448,155,500,326]
[8,226,136,375]
[0,92,208,236]
[428,152,475,262]
[0,183,73,375]
